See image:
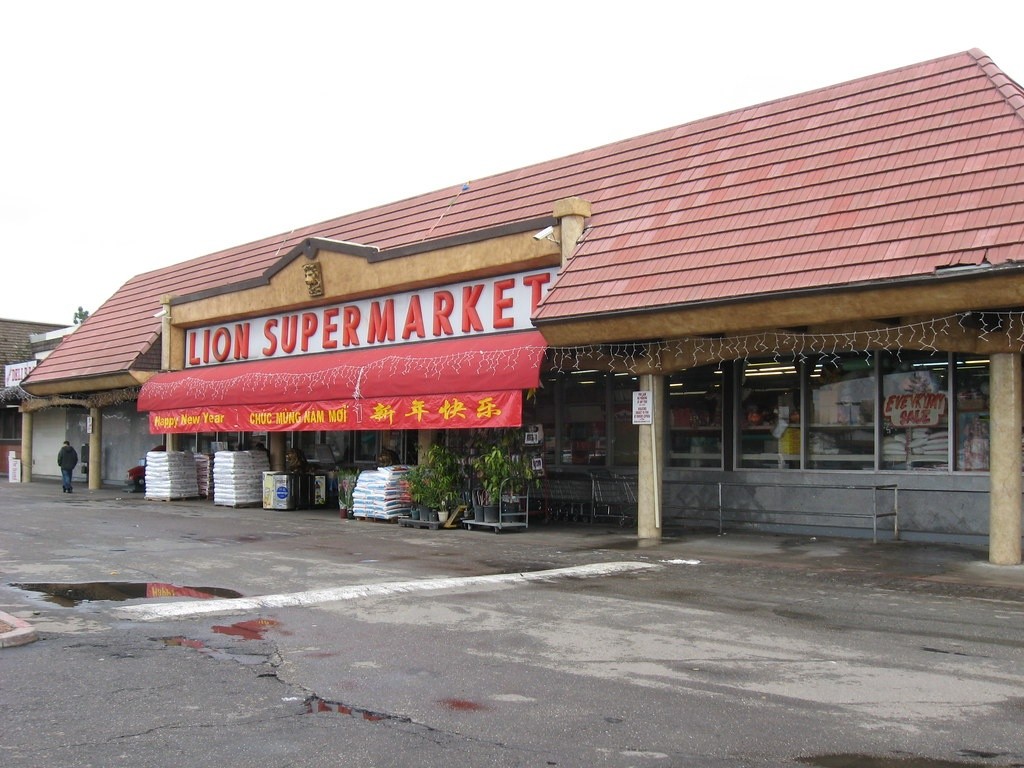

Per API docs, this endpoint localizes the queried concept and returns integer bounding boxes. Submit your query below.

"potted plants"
[472,447,540,524]
[337,468,359,519]
[403,444,460,522]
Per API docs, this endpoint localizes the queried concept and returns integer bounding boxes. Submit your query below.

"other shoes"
[68,487,73,493]
[63,486,66,492]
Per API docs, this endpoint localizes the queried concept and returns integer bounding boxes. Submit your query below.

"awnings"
[136,329,547,413]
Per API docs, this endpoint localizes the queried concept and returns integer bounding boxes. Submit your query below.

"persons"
[57,440,77,494]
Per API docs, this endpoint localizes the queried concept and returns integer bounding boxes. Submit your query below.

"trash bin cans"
[261,470,296,510]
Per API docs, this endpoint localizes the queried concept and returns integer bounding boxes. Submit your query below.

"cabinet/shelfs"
[542,351,989,469]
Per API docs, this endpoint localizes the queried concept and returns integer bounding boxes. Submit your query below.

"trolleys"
[462,475,531,534]
[527,470,638,526]
